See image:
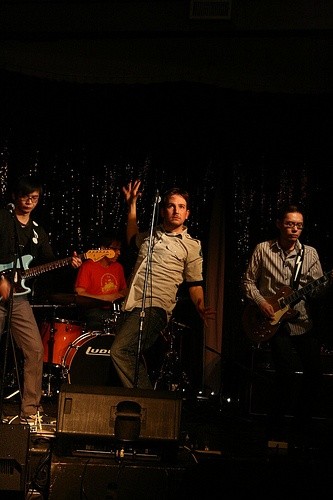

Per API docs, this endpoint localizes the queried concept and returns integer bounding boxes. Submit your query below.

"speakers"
[48,453,196,500]
[56,385,182,441]
[41,318,114,386]
[247,348,333,420]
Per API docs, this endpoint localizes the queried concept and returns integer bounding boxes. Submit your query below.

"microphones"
[7,203,16,213]
[151,196,162,206]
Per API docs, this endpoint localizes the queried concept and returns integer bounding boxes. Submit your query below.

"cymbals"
[50,293,97,302]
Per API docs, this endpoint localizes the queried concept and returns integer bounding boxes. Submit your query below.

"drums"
[60,330,123,387]
[40,314,88,381]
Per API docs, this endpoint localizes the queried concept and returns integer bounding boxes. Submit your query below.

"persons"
[110,177,217,391]
[0,175,82,425]
[241,205,324,409]
[74,239,130,303]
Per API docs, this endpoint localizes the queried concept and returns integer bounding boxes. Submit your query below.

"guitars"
[0,246,115,301]
[237,271,333,344]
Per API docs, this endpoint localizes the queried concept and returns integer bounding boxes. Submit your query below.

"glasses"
[283,221,304,229]
[20,195,40,202]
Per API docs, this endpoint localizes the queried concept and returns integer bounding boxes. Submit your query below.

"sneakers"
[20,411,57,425]
